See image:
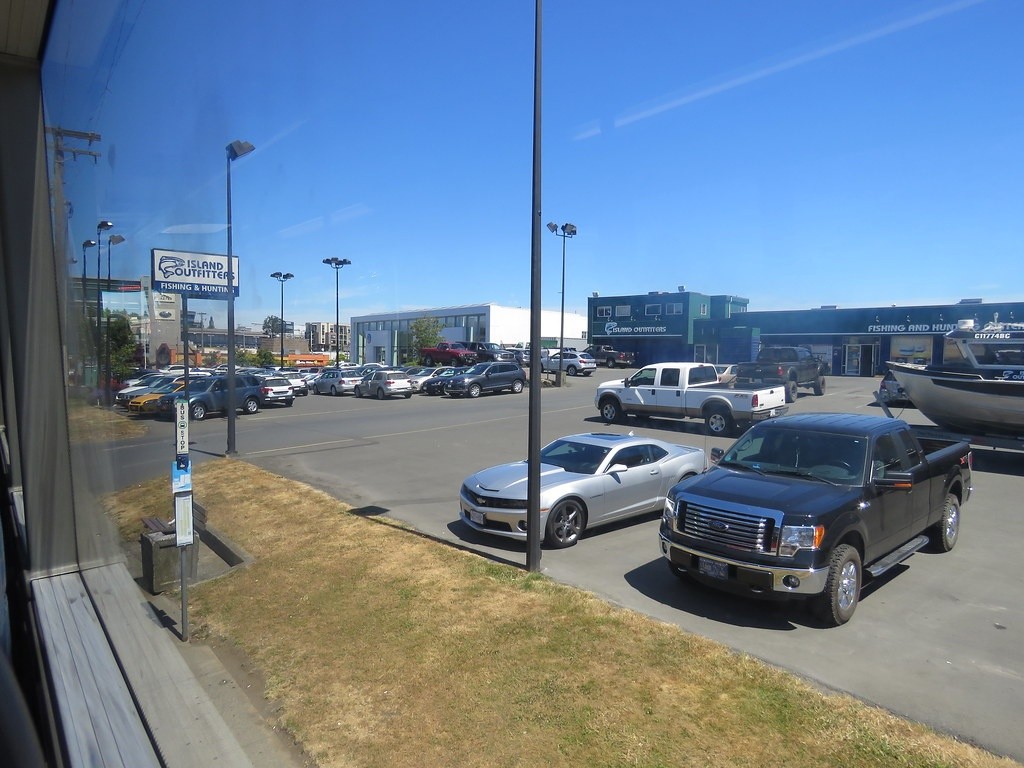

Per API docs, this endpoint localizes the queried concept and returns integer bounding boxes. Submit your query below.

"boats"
[882,319,1024,438]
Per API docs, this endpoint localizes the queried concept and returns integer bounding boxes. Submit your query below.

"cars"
[116,365,294,414]
[276,363,448,396]
[711,364,738,389]
[879,371,911,402]
[458,432,709,548]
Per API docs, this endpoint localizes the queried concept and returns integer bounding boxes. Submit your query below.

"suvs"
[445,362,527,398]
[157,374,264,421]
[508,345,634,376]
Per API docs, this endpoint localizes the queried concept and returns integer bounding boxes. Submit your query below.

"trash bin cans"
[140,527,199,593]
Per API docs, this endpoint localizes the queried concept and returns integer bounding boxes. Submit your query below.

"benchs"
[140,498,208,537]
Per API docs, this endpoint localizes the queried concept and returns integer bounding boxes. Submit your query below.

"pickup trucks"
[594,360,789,435]
[735,347,826,402]
[657,411,971,627]
[421,341,516,367]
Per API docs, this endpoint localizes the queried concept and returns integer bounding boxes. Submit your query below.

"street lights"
[226,140,257,455]
[322,258,352,367]
[83,239,95,316]
[547,222,577,386]
[105,235,124,404]
[96,220,112,398]
[271,272,294,371]
